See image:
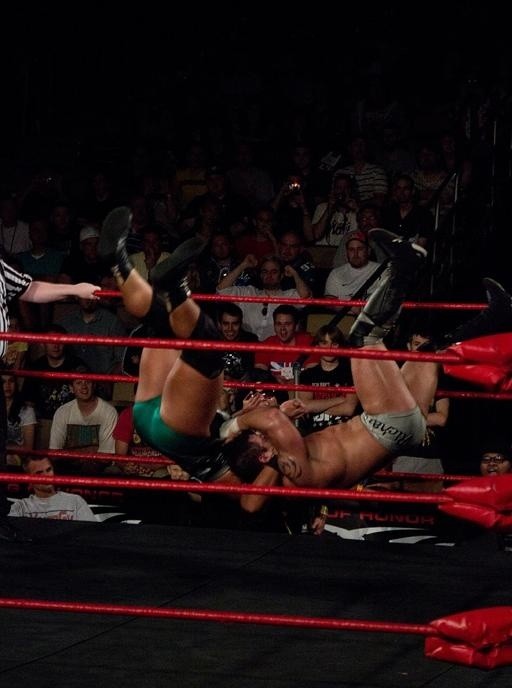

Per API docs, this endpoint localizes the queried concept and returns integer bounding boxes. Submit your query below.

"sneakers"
[97,206,135,260]
[369,229,428,268]
[484,277,511,323]
[147,235,206,289]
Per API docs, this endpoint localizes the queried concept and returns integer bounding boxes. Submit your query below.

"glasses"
[481,456,506,464]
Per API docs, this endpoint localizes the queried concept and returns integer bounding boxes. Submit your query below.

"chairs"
[1,241,396,525]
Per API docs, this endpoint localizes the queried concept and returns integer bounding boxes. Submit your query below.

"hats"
[79,227,99,241]
[344,232,366,248]
